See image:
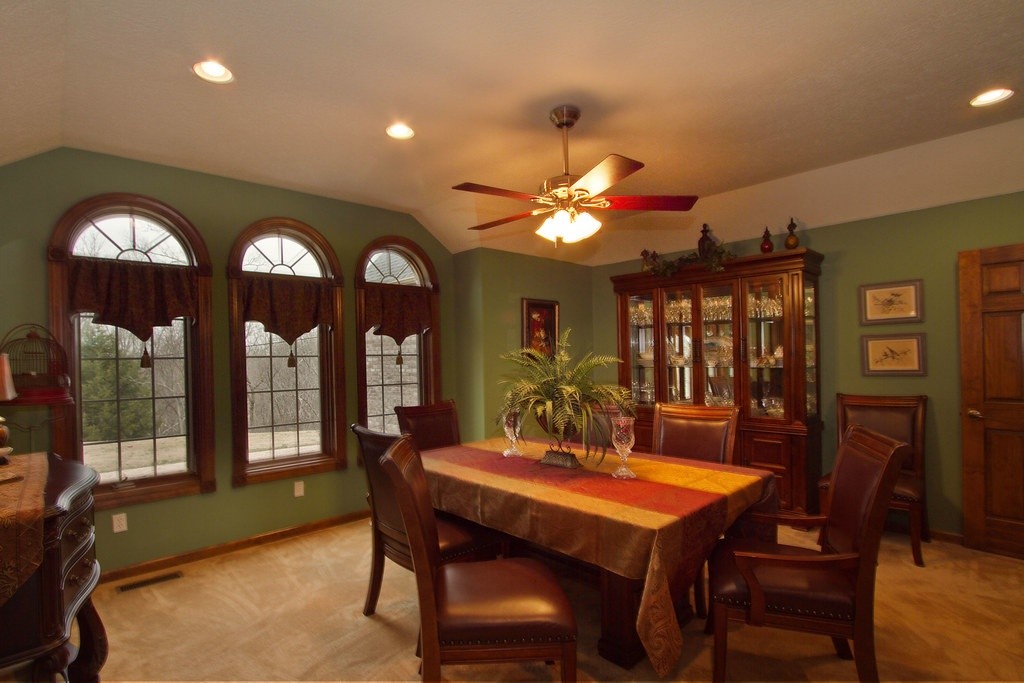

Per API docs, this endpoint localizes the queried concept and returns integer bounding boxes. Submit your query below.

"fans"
[451,105,698,231]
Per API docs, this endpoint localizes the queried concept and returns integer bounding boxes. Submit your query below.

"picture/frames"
[858,279,924,326]
[859,332,929,377]
[521,298,560,367]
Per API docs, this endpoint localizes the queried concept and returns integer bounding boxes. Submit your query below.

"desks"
[419,436,779,683]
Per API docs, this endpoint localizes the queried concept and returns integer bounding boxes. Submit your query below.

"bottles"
[760,226,773,253]
[784,218,799,249]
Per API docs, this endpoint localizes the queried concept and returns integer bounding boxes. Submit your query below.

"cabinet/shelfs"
[609,248,824,530]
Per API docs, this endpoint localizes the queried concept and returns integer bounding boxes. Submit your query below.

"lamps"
[536,200,602,248]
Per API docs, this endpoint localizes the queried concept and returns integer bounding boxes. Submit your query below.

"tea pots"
[669,355,689,366]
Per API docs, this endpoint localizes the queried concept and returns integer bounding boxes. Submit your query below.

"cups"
[761,397,784,409]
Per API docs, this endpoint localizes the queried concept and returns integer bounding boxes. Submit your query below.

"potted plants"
[492,327,638,469]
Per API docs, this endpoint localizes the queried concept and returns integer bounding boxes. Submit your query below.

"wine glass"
[611,416,637,478]
[502,407,524,457]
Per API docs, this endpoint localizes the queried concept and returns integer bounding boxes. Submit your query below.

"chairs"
[394,395,461,450]
[378,434,578,683]
[652,402,740,464]
[702,424,911,683]
[350,423,501,656]
[819,394,931,567]
